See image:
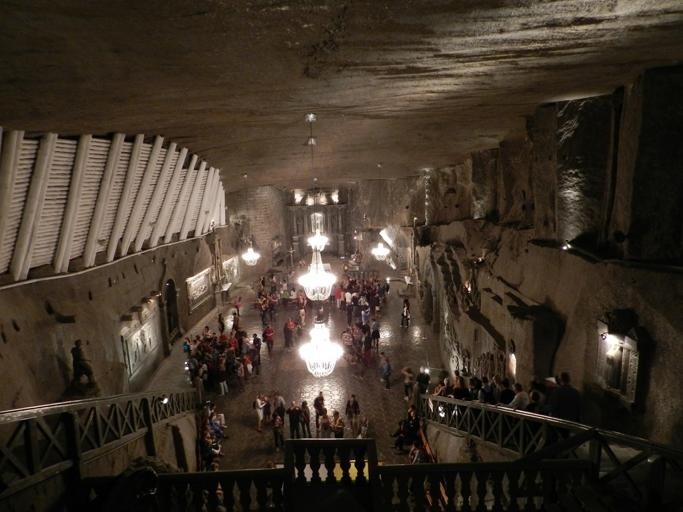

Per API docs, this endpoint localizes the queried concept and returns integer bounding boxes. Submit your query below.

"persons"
[182,311,263,399]
[400,296,410,328]
[252,392,369,453]
[196,400,228,473]
[401,365,583,473]
[330,274,390,355]
[70,339,94,385]
[354,351,367,381]
[255,273,307,351]
[233,294,241,317]
[378,352,393,390]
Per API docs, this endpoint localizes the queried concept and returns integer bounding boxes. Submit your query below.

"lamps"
[241,113,391,377]
